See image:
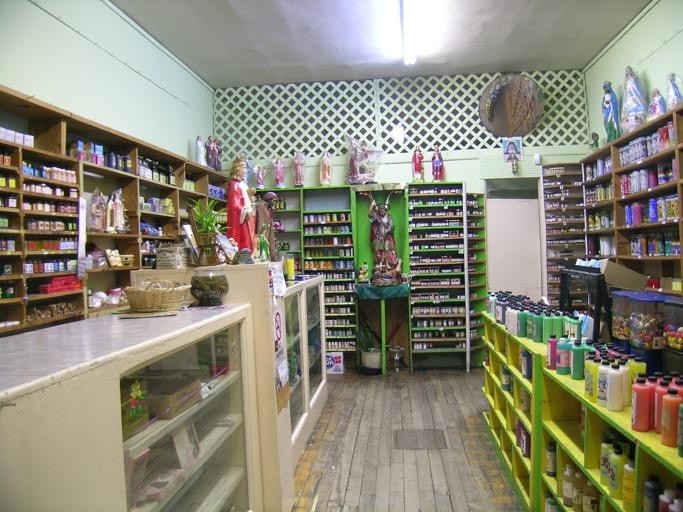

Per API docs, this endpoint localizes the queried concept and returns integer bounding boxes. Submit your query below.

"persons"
[411,143,423,182]
[647,88,666,120]
[600,80,620,143]
[363,189,400,272]
[431,145,443,180]
[271,157,287,188]
[666,71,683,111]
[292,150,305,184]
[346,140,374,178]
[319,151,330,184]
[358,267,367,279]
[195,134,223,172]
[217,155,277,265]
[619,66,645,134]
[90,189,126,234]
[503,141,520,161]
[361,260,368,279]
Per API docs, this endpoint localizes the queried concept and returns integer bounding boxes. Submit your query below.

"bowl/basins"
[360,348,382,369]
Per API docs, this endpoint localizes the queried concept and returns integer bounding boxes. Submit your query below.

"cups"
[190,269,229,306]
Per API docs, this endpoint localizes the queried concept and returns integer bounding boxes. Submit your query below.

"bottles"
[105,150,169,184]
[0,280,15,299]
[59,237,78,250]
[276,241,289,251]
[6,194,18,207]
[87,289,93,309]
[485,290,683,512]
[0,172,17,189]
[41,183,46,194]
[305,213,354,269]
[22,161,77,184]
[70,188,77,198]
[271,195,286,211]
[22,199,79,231]
[23,258,77,275]
[583,121,681,257]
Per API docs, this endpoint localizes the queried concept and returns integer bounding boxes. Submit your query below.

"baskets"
[123,277,192,313]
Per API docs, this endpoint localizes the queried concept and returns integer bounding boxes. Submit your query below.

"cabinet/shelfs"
[0,306,260,512]
[271,275,329,473]
[540,104,683,376]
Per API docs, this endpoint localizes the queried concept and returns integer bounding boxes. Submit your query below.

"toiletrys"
[484,289,683,512]
